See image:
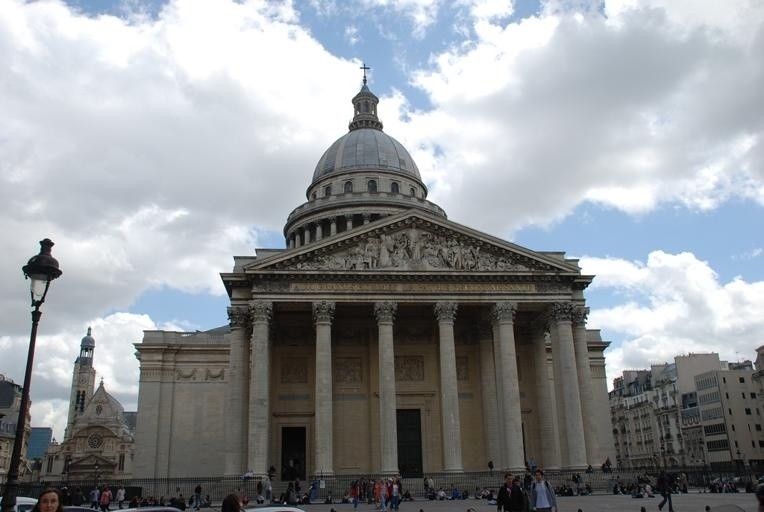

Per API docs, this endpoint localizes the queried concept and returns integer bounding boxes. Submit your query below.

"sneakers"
[658,505,662,510]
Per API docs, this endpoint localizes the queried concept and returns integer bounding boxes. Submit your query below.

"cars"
[687,474,764,487]
[655,470,688,494]
[0,496,306,512]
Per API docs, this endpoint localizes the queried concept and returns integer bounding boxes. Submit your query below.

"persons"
[292,221,519,273]
[27,469,763,511]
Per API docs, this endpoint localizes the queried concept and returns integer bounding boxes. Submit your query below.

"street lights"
[659,442,668,471]
[93,459,99,488]
[64,455,72,484]
[736,448,742,487]
[0,238,62,511]
